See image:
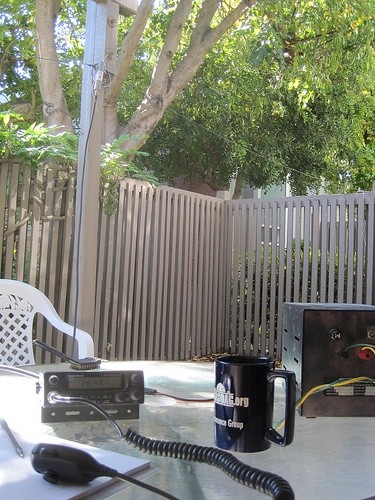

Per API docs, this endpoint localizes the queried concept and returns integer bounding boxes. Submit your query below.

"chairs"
[0,279,95,367]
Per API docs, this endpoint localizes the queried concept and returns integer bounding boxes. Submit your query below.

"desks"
[0,359,375,500]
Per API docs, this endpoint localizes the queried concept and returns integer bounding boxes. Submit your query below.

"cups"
[214,356,296,454]
